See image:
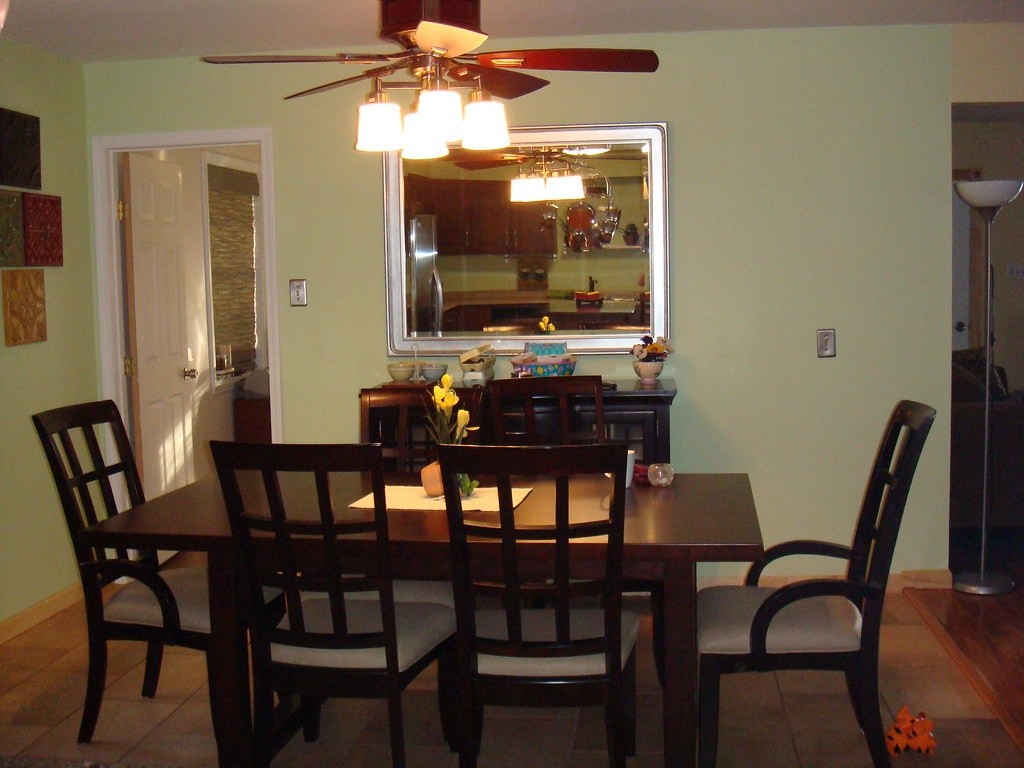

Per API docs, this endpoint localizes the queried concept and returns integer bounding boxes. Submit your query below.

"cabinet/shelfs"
[472,179,558,257]
[357,377,677,475]
[405,173,473,257]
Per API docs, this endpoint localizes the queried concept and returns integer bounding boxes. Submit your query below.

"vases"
[632,360,665,385]
[539,330,551,336]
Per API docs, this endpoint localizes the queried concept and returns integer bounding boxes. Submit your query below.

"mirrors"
[379,121,671,358]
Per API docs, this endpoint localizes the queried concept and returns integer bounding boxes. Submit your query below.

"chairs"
[31,399,289,746]
[437,440,638,768]
[693,400,939,768]
[206,439,460,768]
[360,386,510,757]
[950,348,1024,531]
[486,374,664,760]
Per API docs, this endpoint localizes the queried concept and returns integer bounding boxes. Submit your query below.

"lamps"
[510,156,586,202]
[356,51,511,161]
[953,178,1024,600]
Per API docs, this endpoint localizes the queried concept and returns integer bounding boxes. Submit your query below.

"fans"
[202,0,660,99]
[453,143,646,172]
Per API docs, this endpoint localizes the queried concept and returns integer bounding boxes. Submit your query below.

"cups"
[625,450,635,489]
[648,464,674,486]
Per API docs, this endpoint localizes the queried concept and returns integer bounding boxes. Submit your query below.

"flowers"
[538,316,556,331]
[632,335,676,362]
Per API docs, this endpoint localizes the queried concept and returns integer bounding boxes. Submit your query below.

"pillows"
[951,362,999,403]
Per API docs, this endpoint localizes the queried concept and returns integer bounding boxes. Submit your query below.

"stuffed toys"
[887,704,937,758]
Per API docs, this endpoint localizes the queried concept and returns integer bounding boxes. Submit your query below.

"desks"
[77,471,764,768]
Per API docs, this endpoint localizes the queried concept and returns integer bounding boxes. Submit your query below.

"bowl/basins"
[387,362,415,381]
[421,364,448,381]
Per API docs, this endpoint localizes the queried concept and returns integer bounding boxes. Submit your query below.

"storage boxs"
[461,366,496,389]
[459,342,496,372]
